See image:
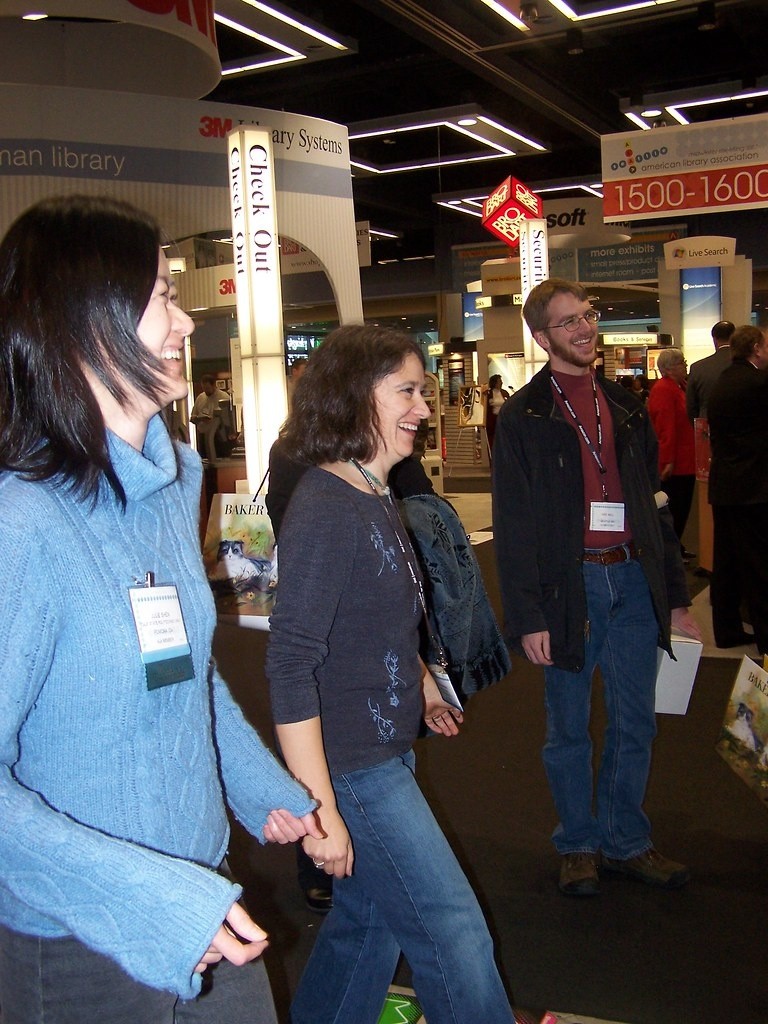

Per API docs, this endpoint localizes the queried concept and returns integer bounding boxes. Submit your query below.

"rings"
[434,716,441,721]
[313,857,326,868]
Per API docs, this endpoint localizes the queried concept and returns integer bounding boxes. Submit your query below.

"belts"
[582,544,634,565]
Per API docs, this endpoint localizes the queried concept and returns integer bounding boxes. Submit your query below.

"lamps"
[629,89,643,110]
[519,4,539,21]
[697,2,716,30]
[566,28,584,54]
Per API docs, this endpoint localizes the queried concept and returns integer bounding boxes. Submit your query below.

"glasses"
[672,360,688,365]
[538,309,601,331]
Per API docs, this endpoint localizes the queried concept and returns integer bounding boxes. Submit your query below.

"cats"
[214,539,271,579]
[726,702,764,754]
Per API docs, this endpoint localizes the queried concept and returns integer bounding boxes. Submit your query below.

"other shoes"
[716,633,757,648]
[682,549,696,565]
[304,888,333,912]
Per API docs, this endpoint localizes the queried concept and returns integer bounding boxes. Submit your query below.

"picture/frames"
[457,385,487,427]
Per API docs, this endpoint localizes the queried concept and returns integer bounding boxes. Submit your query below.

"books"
[717,651,767,809]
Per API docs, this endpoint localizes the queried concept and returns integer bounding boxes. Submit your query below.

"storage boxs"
[655,626,703,716]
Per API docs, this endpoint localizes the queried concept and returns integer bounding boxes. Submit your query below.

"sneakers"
[559,852,600,896]
[602,848,690,888]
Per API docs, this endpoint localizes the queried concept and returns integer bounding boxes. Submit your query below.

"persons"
[683,323,739,419]
[268,319,518,1023]
[704,324,768,656]
[189,373,230,459]
[642,345,698,567]
[620,373,650,405]
[288,358,308,418]
[487,271,689,888]
[2,188,331,1024]
[483,374,510,470]
